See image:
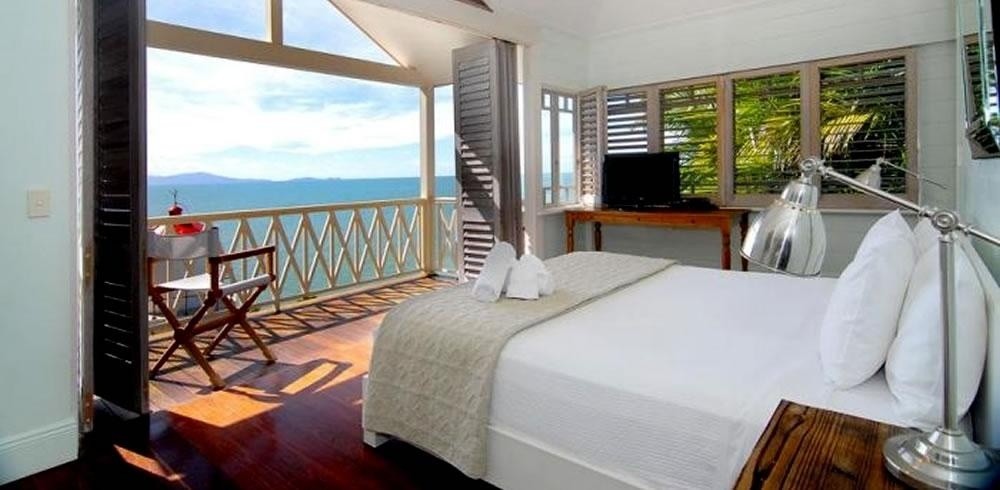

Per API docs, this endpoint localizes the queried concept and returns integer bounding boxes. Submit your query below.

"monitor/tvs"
[602,151,680,211]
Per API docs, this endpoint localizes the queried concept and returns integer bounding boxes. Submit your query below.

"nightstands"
[725,399,940,489]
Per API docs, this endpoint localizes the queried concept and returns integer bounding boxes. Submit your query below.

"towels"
[471,241,557,302]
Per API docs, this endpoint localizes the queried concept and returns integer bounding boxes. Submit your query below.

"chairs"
[149,228,278,389]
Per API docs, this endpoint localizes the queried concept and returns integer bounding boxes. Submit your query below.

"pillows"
[819,208,919,394]
[883,205,988,429]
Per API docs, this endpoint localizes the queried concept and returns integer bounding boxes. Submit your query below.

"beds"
[362,251,998,490]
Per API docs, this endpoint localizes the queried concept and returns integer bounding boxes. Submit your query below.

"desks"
[564,207,749,271]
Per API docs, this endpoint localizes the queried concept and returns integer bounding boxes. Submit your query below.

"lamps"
[737,156,1000,490]
[848,150,952,229]
